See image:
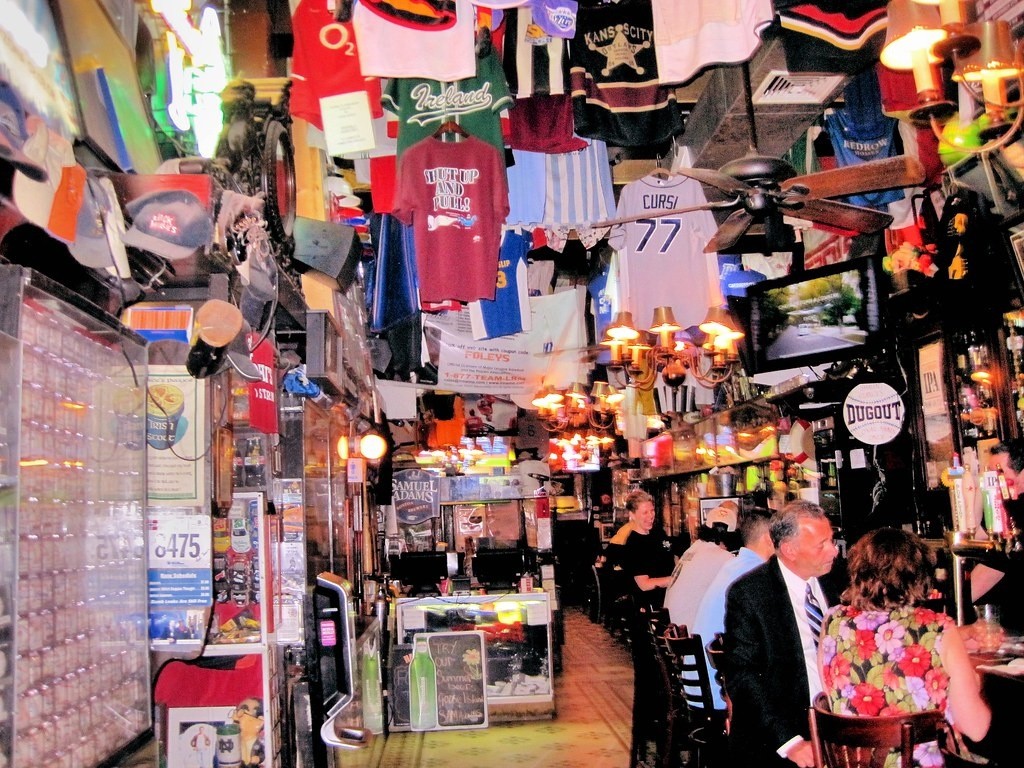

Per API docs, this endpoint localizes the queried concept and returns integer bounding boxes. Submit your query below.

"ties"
[804,582,824,652]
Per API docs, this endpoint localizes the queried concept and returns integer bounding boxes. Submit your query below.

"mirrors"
[259,120,298,241]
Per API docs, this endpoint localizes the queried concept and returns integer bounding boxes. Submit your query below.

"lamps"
[881,0,1024,152]
[532,382,625,434]
[951,552,1006,627]
[601,306,745,398]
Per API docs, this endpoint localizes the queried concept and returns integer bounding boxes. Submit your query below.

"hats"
[120,189,212,260]
[705,507,738,533]
[0,78,132,279]
[185,299,261,380]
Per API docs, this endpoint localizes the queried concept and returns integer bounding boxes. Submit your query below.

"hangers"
[432,105,470,140]
[648,154,675,177]
[946,166,971,196]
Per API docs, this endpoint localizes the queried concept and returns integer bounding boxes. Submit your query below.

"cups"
[975,606,999,659]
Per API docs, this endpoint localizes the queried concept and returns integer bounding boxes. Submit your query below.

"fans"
[590,63,926,253]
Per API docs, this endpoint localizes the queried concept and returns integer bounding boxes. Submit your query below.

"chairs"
[613,594,944,768]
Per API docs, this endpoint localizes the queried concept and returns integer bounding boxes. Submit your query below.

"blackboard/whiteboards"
[392,650,415,726]
[429,635,484,726]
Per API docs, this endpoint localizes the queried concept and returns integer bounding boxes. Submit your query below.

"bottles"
[409,637,436,729]
[363,659,382,735]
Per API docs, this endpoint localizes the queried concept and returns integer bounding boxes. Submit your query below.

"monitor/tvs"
[470,547,524,583]
[400,551,448,585]
[747,254,885,373]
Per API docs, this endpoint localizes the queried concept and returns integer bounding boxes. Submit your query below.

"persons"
[724,500,850,768]
[618,490,680,613]
[959,437,1024,637]
[662,499,780,712]
[816,527,992,768]
